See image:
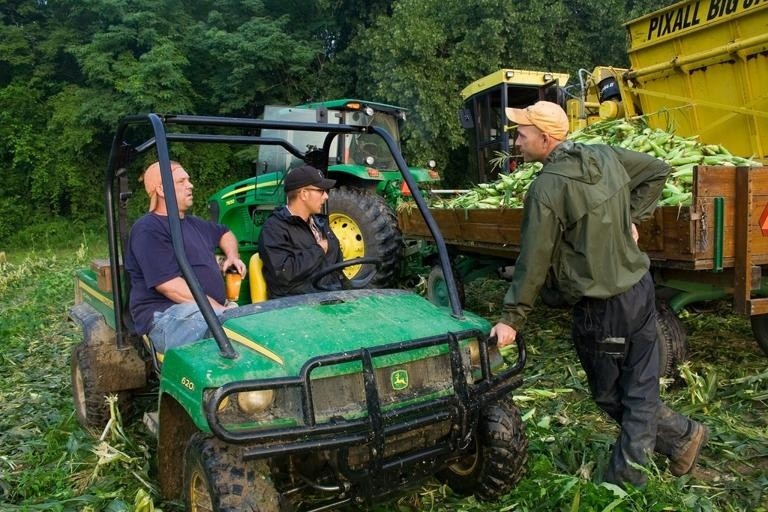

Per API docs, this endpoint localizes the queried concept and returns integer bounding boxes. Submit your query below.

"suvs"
[66,115,526,512]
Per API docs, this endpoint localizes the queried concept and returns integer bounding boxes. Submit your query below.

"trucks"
[208,98,768,390]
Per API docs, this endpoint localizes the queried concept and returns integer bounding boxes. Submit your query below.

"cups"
[225,270,241,301]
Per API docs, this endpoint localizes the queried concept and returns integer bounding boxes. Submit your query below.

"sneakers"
[670,421,708,476]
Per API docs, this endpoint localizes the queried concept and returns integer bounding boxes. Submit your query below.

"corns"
[566,114,763,210]
[399,161,543,212]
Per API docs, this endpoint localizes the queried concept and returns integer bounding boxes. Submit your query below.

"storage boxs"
[90,258,125,294]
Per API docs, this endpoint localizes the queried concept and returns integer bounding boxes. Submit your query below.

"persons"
[256,165,351,302]
[489,100,710,492]
[123,158,248,355]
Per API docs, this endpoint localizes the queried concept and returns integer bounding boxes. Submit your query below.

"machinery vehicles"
[444,66,641,194]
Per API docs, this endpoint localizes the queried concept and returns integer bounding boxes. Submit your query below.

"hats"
[283,164,336,190]
[143,161,181,212]
[505,102,571,140]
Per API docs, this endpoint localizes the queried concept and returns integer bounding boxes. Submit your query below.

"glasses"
[304,186,324,194]
